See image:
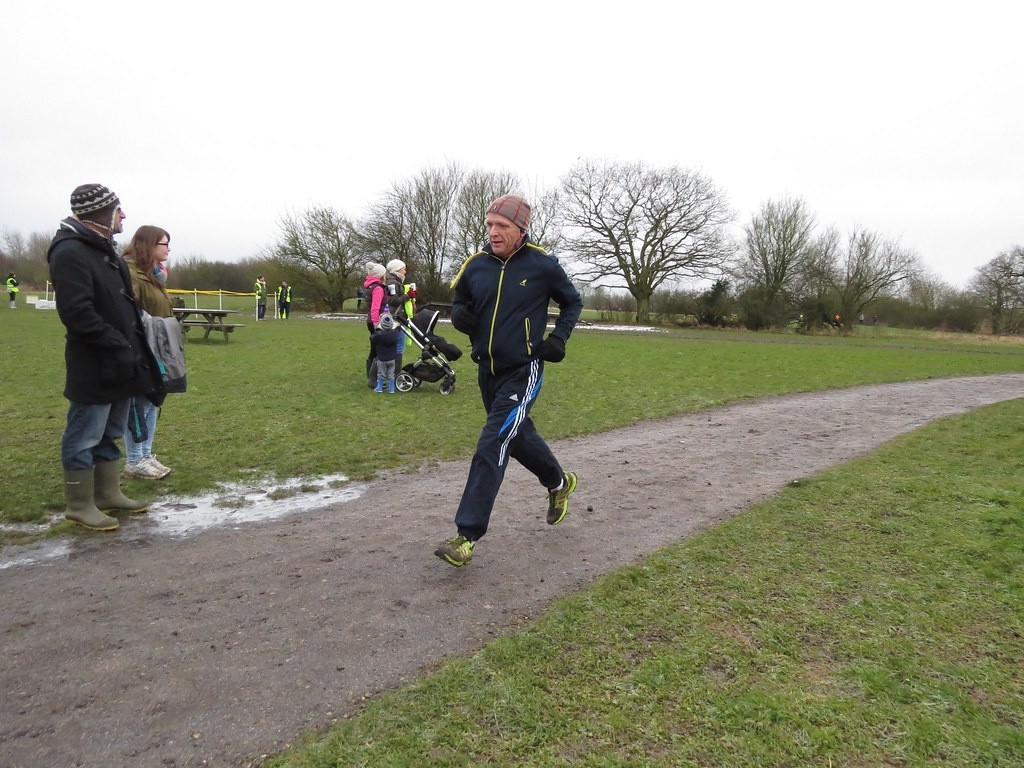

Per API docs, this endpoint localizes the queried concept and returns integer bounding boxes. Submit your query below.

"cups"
[410,282,416,292]
[388,284,396,295]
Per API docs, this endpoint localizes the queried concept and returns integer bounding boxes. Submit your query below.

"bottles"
[384,304,390,314]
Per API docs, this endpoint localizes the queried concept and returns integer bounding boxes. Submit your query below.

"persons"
[275,280,293,319]
[356,259,419,394]
[119,225,178,479]
[434,194,584,566]
[6,272,19,309]
[254,275,267,320]
[46,183,166,530]
[800,313,878,326]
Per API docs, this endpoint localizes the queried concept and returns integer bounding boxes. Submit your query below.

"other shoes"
[121,454,170,480]
[9,300,16,309]
[368,376,388,389]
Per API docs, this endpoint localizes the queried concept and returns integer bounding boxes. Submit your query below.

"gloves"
[407,288,417,298]
[451,301,478,335]
[539,334,566,362]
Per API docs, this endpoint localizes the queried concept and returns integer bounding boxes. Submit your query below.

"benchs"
[179,320,246,343]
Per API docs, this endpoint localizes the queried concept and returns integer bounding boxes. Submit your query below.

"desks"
[172,308,238,344]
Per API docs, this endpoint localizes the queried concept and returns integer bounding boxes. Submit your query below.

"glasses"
[156,241,170,249]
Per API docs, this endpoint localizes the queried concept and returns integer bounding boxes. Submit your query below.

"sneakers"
[545,470,577,525]
[434,535,476,567]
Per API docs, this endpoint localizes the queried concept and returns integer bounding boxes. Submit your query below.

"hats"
[387,259,406,272]
[486,194,532,233]
[366,262,386,279]
[9,272,15,276]
[380,314,393,330]
[70,183,122,270]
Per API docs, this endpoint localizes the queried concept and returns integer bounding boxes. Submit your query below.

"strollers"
[394,301,457,395]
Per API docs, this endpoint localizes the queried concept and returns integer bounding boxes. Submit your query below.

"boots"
[94,458,151,515]
[387,378,395,394]
[63,468,120,531]
[373,379,383,393]
[366,359,373,377]
[395,353,403,378]
